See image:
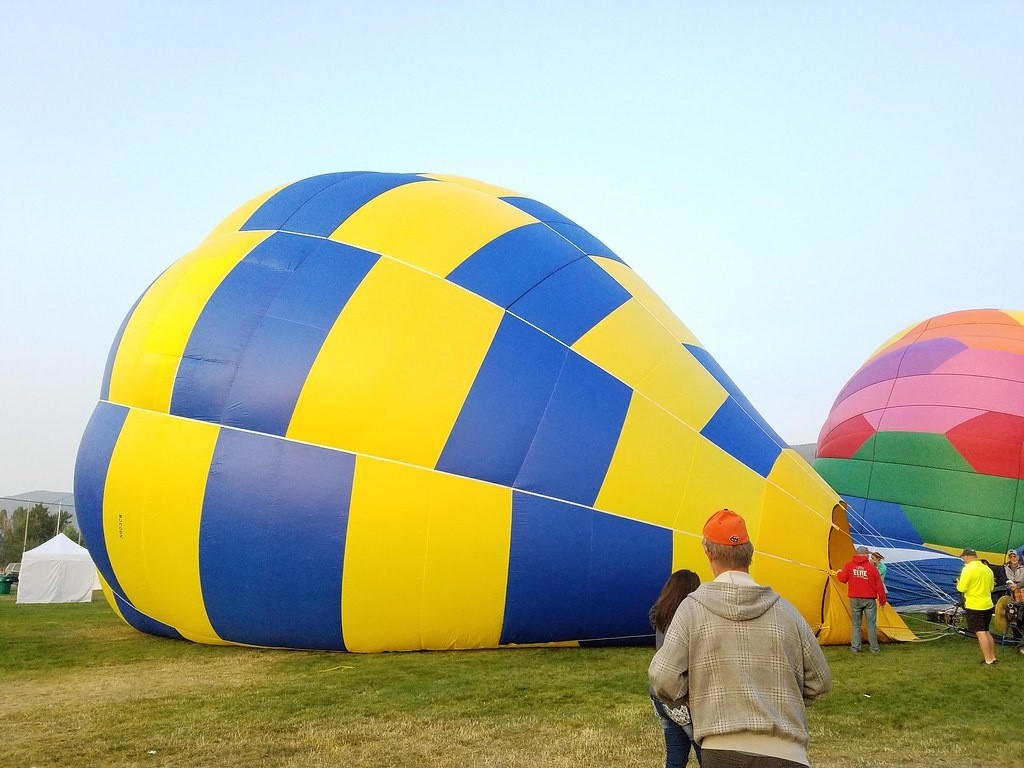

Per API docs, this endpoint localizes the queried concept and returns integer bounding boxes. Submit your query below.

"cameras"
[953,579,958,583]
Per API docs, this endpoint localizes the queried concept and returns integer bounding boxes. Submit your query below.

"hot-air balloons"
[73,166,977,656]
[811,308,1024,614]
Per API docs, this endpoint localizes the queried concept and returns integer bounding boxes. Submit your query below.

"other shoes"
[853,648,860,652]
[980,659,998,665]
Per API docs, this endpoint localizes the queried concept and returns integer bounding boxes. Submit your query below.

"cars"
[5,562,21,581]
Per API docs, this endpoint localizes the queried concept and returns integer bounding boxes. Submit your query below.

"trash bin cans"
[0,573,15,594]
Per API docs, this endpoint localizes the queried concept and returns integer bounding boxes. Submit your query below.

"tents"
[15,533,103,604]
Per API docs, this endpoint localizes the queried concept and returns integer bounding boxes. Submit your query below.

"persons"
[837,546,886,653]
[956,548,999,666]
[869,552,889,594]
[980,549,1024,617]
[649,569,702,768]
[648,509,832,768]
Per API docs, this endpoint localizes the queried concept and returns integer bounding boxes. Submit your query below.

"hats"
[857,547,873,554]
[1008,549,1017,555]
[959,549,976,558]
[703,508,750,546]
